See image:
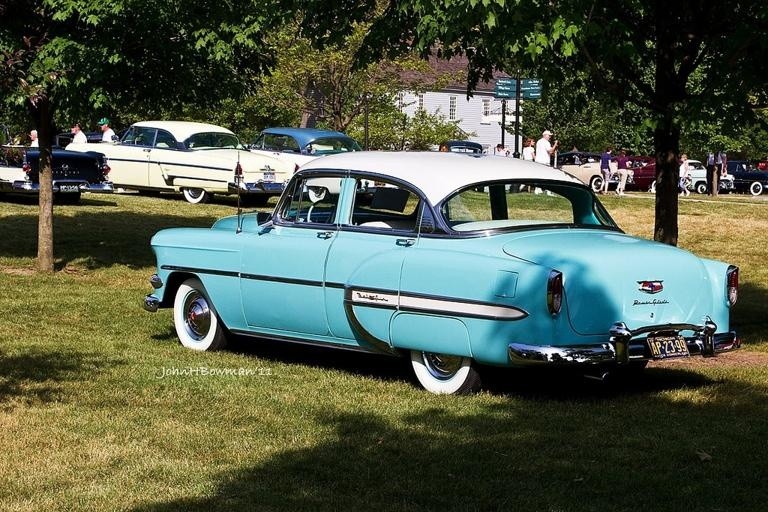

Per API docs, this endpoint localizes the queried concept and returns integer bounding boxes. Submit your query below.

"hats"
[542,130,553,136]
[97,117,109,124]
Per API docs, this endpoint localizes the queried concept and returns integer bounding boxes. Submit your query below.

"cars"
[552,151,633,194]
[66,120,301,204]
[724,161,768,196]
[0,144,114,194]
[247,127,361,153]
[626,155,657,191]
[144,150,742,400]
[440,141,482,156]
[652,158,737,194]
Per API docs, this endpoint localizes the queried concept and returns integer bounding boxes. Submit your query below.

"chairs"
[371,187,409,213]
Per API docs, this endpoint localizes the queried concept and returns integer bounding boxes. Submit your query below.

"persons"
[505,150,512,157]
[678,153,690,197]
[97,117,115,142]
[69,123,88,143]
[439,143,449,152]
[707,148,725,196]
[598,147,613,195]
[519,138,535,193]
[614,150,631,195]
[534,130,560,197]
[30,130,40,147]
[496,144,507,158]
[717,153,728,193]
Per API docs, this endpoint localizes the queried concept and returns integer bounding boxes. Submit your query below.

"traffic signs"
[520,78,542,100]
[493,77,517,99]
